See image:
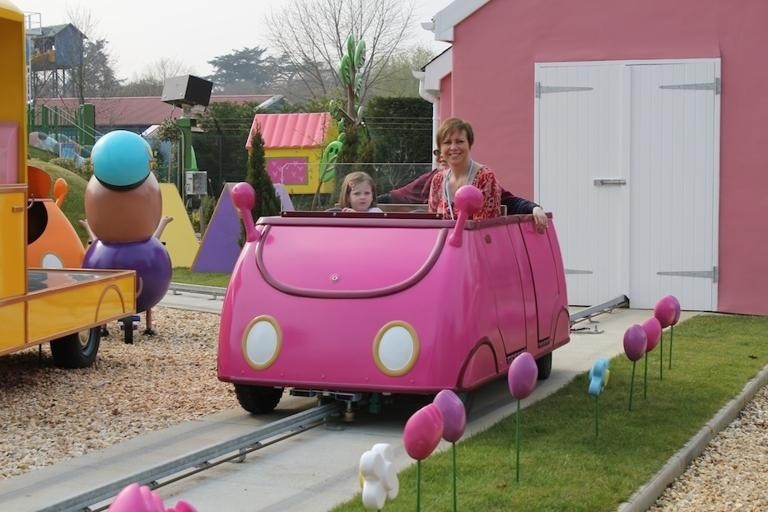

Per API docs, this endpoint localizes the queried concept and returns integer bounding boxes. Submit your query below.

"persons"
[339,171,386,212]
[376,115,548,235]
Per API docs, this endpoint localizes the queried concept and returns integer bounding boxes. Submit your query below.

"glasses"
[434,149,441,156]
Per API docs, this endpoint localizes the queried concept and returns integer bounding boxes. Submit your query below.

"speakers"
[160,74,213,112]
